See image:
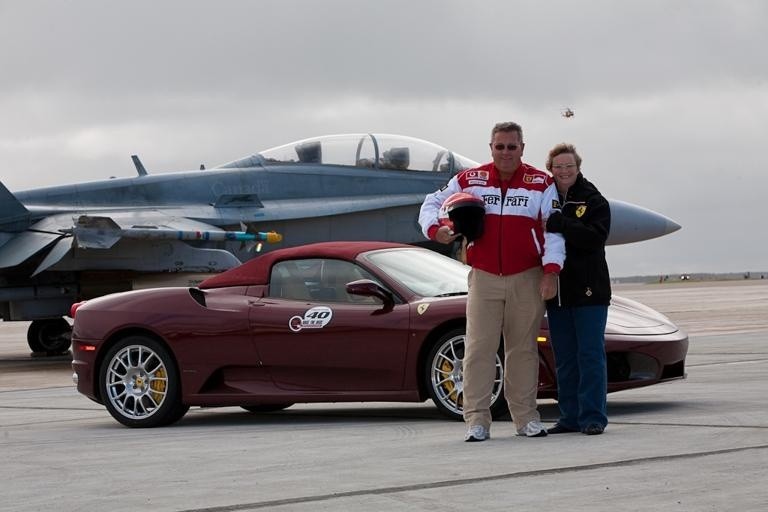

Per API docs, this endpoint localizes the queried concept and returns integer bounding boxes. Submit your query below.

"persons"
[540,141,614,436]
[417,120,567,442]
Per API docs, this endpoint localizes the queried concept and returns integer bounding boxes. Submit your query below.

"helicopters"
[560,107,576,117]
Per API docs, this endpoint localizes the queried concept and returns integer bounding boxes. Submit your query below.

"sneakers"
[465,424,491,441]
[547,424,582,434]
[513,419,547,438]
[584,422,604,435]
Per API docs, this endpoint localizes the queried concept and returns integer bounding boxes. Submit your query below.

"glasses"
[551,162,577,171]
[491,142,521,151]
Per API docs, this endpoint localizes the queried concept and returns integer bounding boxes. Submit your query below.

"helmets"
[438,191,486,242]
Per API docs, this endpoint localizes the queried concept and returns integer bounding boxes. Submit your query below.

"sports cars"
[70,240,688,428]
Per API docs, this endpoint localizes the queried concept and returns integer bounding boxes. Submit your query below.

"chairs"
[275,261,378,305]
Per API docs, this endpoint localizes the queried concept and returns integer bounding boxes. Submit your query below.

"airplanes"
[0,133,682,357]
